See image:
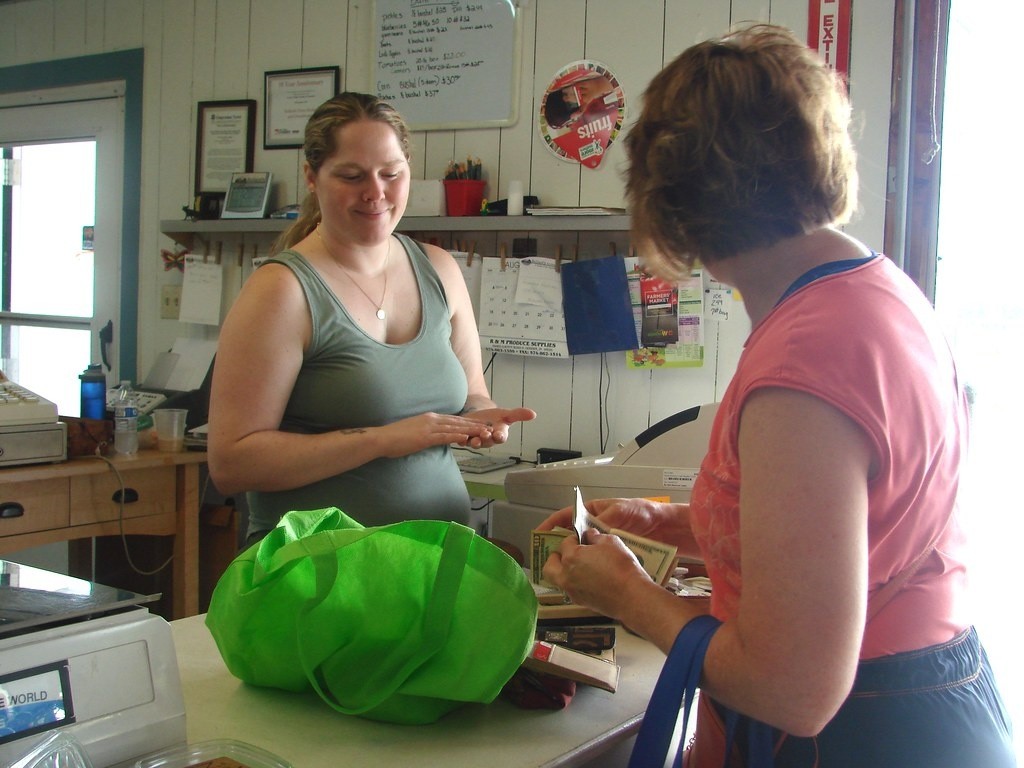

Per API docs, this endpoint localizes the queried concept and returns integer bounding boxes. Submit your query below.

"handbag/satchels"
[205,506,540,726]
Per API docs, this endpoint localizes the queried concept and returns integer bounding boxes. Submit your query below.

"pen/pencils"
[445,154,482,180]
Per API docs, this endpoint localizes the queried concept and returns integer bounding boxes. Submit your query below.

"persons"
[208,92,535,547]
[537,26,1018,768]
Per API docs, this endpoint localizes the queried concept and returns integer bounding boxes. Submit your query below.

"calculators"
[458,456,515,475]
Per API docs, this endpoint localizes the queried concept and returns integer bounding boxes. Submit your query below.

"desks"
[0,444,211,621]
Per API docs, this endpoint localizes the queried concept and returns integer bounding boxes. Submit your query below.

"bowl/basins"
[135,737,293,768]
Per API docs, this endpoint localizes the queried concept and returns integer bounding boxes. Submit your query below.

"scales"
[0,557,188,768]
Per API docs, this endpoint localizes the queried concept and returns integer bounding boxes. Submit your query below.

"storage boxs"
[198,502,240,618]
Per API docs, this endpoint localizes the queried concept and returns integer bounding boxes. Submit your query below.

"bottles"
[114,380,139,454]
[78,363,107,420]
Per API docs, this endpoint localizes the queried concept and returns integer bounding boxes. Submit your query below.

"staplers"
[488,195,540,216]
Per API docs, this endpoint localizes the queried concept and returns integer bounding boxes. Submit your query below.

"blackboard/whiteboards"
[372,0,521,131]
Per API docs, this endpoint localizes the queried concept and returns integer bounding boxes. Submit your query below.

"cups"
[152,408,188,453]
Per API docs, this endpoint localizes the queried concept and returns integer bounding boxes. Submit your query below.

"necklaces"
[317,222,391,321]
